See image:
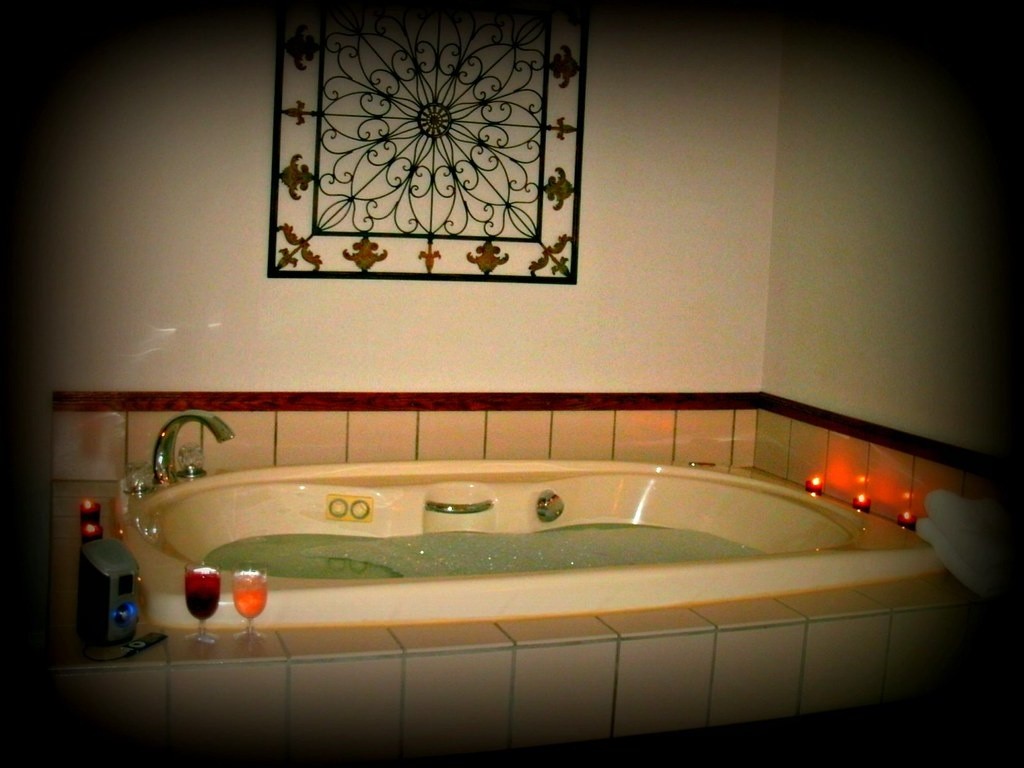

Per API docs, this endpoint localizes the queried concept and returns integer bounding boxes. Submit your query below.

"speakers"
[76,536,138,646]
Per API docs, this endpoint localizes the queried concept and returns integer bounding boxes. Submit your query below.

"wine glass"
[184,560,221,645]
[232,560,269,643]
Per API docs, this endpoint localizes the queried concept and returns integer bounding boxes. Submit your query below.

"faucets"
[151,409,235,490]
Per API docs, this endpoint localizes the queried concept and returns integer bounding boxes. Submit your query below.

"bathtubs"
[118,461,952,626]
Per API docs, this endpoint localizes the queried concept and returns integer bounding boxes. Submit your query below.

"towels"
[916,489,1020,605]
